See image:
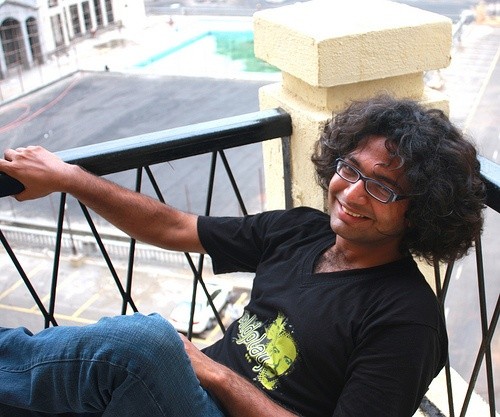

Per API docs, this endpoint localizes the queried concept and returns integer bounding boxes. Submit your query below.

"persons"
[0,93,487,417]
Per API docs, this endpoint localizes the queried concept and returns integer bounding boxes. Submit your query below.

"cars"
[170,283,233,334]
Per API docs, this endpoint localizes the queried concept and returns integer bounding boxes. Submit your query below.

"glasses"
[334,158,416,203]
